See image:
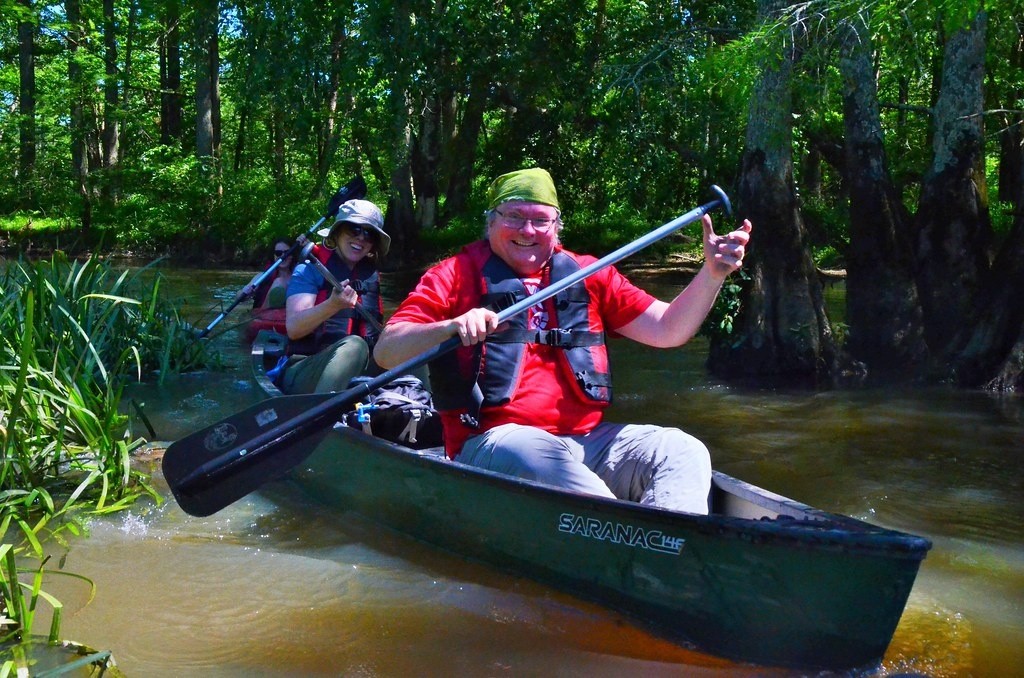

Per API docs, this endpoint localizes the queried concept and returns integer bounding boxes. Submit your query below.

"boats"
[248,359,934,678]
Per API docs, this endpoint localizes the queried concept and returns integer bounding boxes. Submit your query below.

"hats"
[487,167,561,210]
[317,199,392,257]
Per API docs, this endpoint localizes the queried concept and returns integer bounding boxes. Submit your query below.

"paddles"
[296,242,383,334]
[160,182,733,519]
[200,174,368,338]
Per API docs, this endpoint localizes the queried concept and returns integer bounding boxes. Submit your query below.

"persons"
[373,167,752,516]
[235,199,391,395]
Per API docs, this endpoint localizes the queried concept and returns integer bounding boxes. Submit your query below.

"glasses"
[495,209,561,234]
[343,223,380,243]
[273,249,293,256]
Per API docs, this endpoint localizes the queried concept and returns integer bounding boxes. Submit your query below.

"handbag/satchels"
[347,389,444,450]
[345,374,435,416]
